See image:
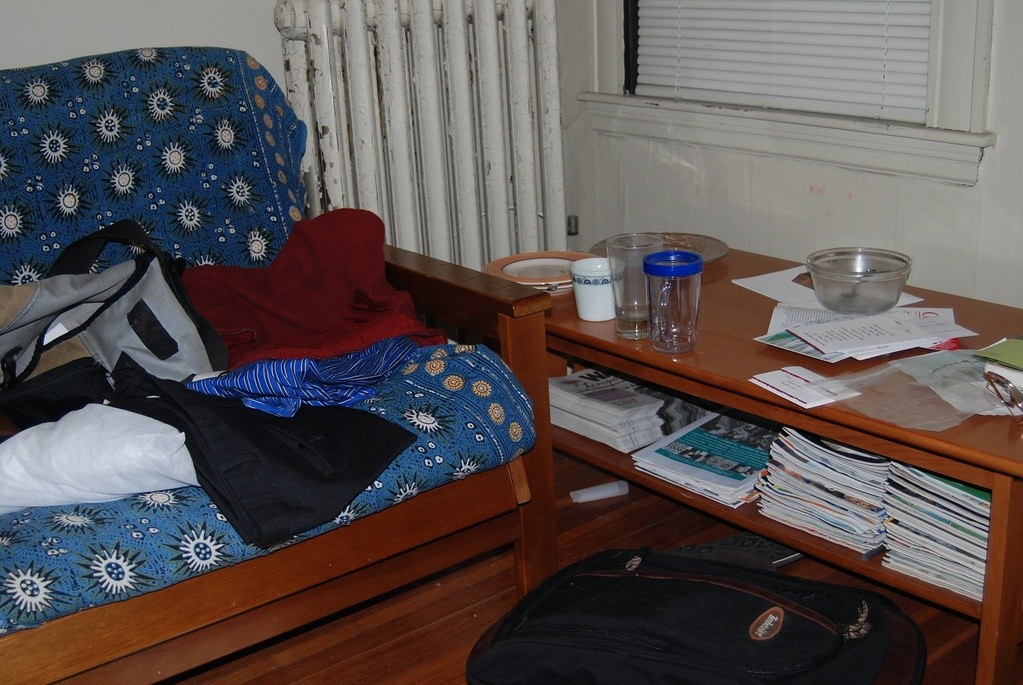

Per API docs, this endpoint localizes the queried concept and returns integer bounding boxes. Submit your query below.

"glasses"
[984,372,1023,425]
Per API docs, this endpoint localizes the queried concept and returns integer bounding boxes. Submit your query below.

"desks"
[541,250,1023,685]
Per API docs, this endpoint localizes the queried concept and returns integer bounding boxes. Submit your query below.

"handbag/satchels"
[0,219,230,382]
[0,346,115,432]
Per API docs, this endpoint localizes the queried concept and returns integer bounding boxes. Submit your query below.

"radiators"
[275,0,568,274]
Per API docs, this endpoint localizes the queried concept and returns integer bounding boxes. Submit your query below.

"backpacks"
[465,546,927,685]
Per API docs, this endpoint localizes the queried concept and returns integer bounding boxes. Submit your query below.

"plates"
[589,231,730,264]
[482,251,601,297]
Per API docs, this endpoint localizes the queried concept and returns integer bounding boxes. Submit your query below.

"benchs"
[0,46,556,685]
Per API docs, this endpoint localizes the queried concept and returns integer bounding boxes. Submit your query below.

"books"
[549,369,991,601]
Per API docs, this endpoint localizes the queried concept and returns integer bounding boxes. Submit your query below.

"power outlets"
[568,216,579,236]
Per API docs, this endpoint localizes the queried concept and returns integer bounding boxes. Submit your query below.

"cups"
[607,233,664,339]
[643,250,703,355]
[570,257,625,321]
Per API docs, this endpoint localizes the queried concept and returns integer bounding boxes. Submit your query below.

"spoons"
[841,268,876,299]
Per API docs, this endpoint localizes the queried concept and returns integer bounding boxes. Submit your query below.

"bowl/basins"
[806,247,913,316]
